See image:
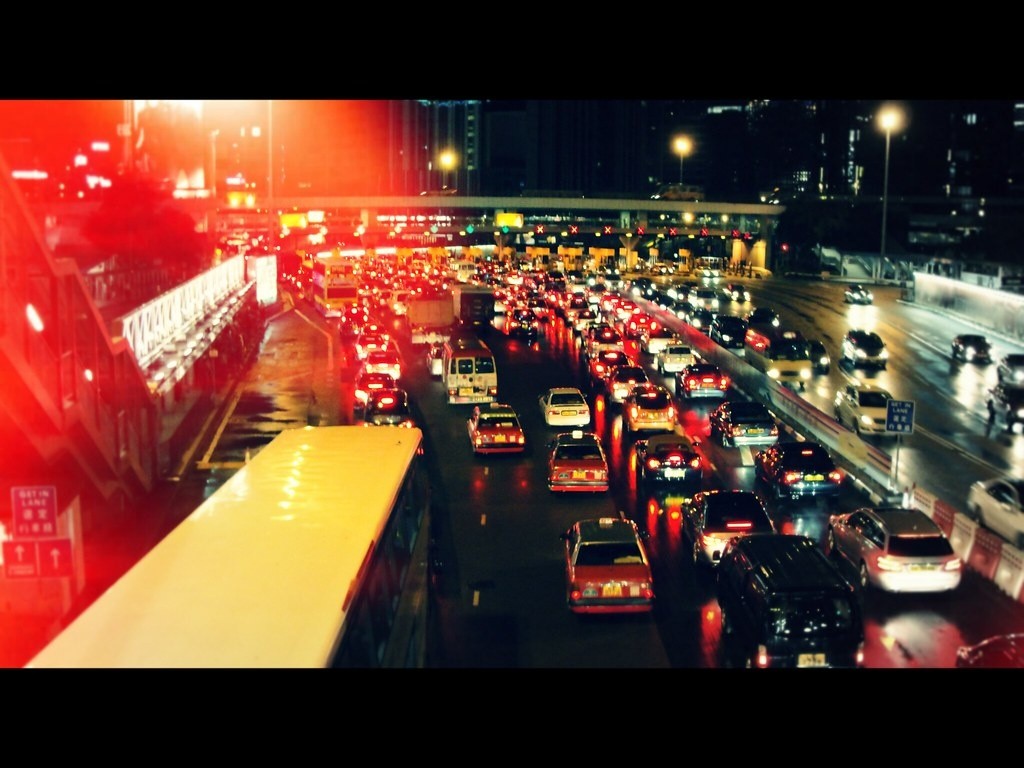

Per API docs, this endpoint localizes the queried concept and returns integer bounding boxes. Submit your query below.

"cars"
[286,258,697,435]
[545,429,611,493]
[419,185,458,196]
[965,475,1024,549]
[755,441,846,503]
[679,488,778,567]
[633,435,703,483]
[711,402,782,448]
[601,262,1024,447]
[956,633,1024,667]
[560,519,656,615]
[828,507,961,594]
[673,363,727,402]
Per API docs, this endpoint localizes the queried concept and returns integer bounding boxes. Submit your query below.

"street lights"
[876,108,897,276]
[674,138,690,183]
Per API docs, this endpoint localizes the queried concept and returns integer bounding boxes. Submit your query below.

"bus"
[313,259,360,317]
[441,339,497,407]
[28,425,438,667]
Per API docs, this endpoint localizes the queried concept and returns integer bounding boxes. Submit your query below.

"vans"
[712,534,867,669]
[652,185,704,202]
